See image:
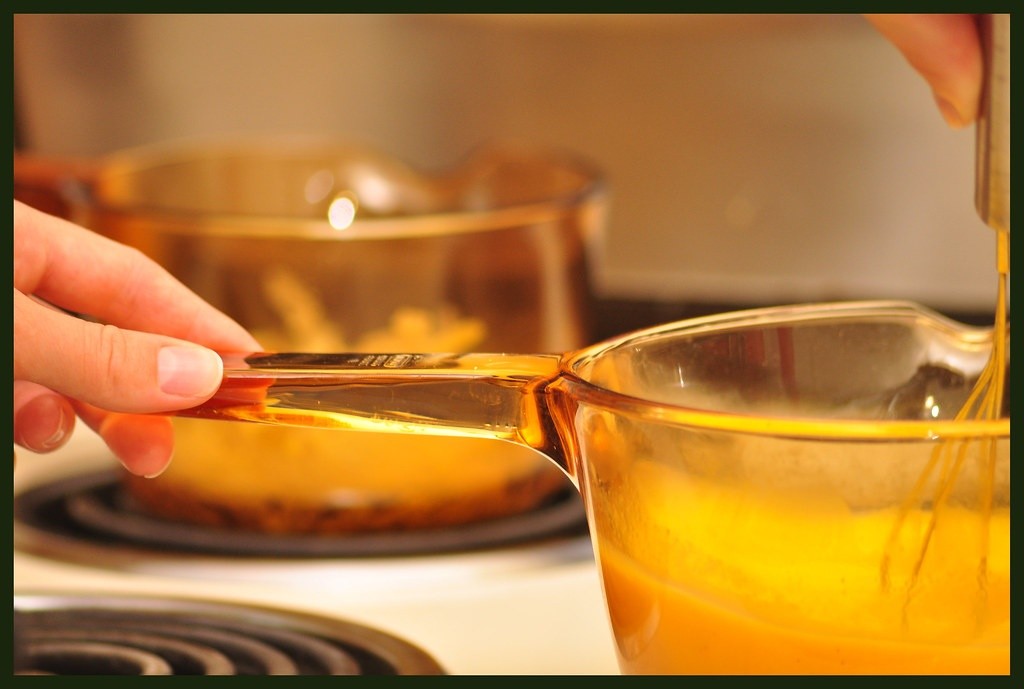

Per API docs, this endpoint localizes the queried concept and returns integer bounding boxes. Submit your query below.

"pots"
[158,302,1011,674]
[13,139,601,535]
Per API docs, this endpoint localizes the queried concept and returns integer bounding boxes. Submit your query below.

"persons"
[864,14,984,130]
[14,200,277,479]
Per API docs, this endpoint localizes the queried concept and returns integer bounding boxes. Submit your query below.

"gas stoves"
[13,347,624,677]
